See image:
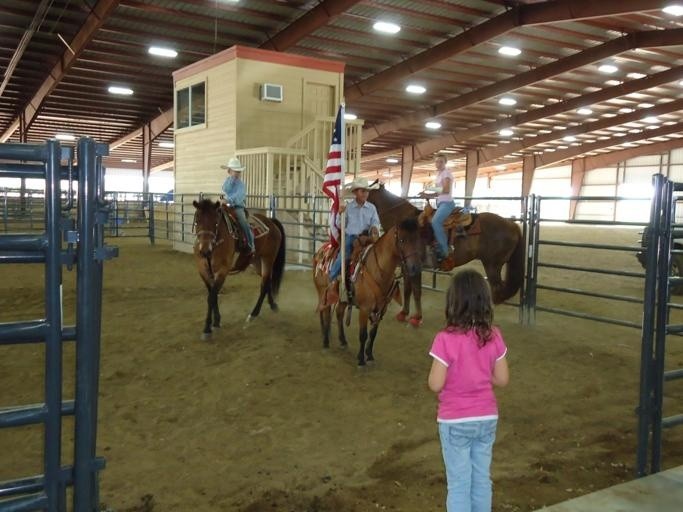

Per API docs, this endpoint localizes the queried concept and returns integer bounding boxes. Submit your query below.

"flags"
[323,100,346,251]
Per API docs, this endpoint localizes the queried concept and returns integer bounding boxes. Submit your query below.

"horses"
[365,178,525,331]
[190,197,286,342]
[312,215,423,368]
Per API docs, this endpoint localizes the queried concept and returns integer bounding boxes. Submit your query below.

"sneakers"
[438,254,449,262]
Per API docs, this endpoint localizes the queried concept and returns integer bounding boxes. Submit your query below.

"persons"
[330,177,381,288]
[419,154,455,262]
[428,270,509,512]
[220,157,256,256]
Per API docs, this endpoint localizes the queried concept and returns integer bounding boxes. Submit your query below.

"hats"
[221,159,246,171]
[351,177,380,191]
